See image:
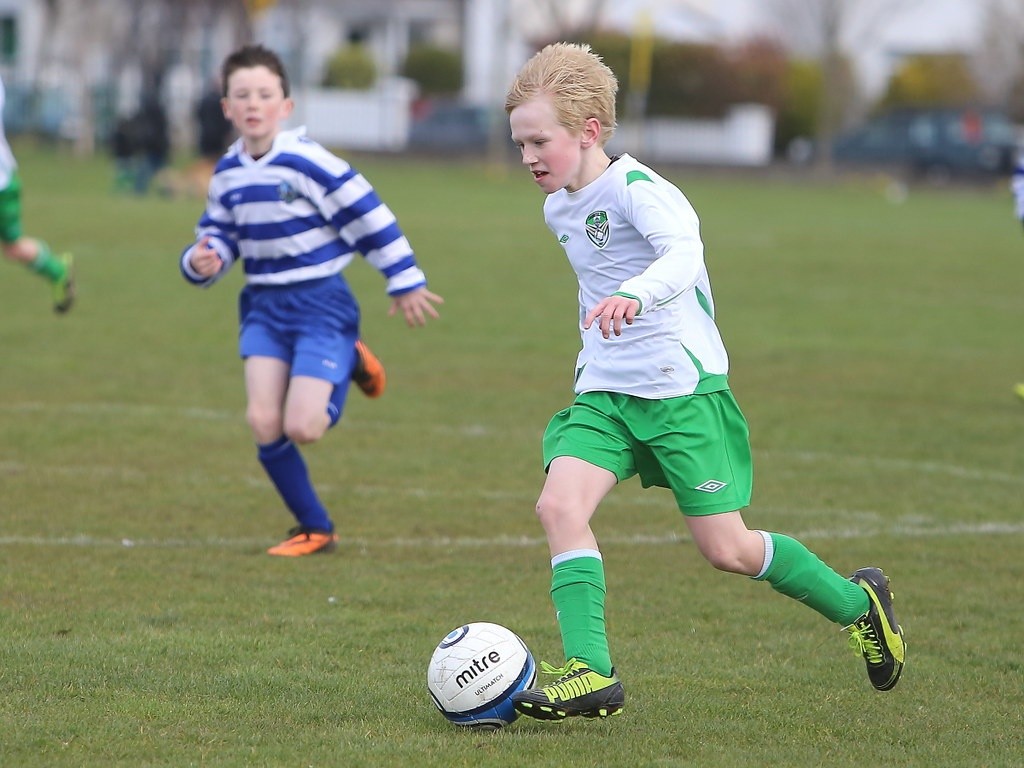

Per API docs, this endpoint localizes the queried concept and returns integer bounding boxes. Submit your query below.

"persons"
[101,14,382,203]
[505,41,911,723]
[0,78,79,314]
[180,43,448,557]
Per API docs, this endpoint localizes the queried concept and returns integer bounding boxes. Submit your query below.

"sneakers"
[840,566,907,691]
[512,657,625,724]
[50,251,77,313]
[353,338,387,399]
[267,527,339,557]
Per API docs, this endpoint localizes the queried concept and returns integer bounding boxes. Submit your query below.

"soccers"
[426,622,536,730]
